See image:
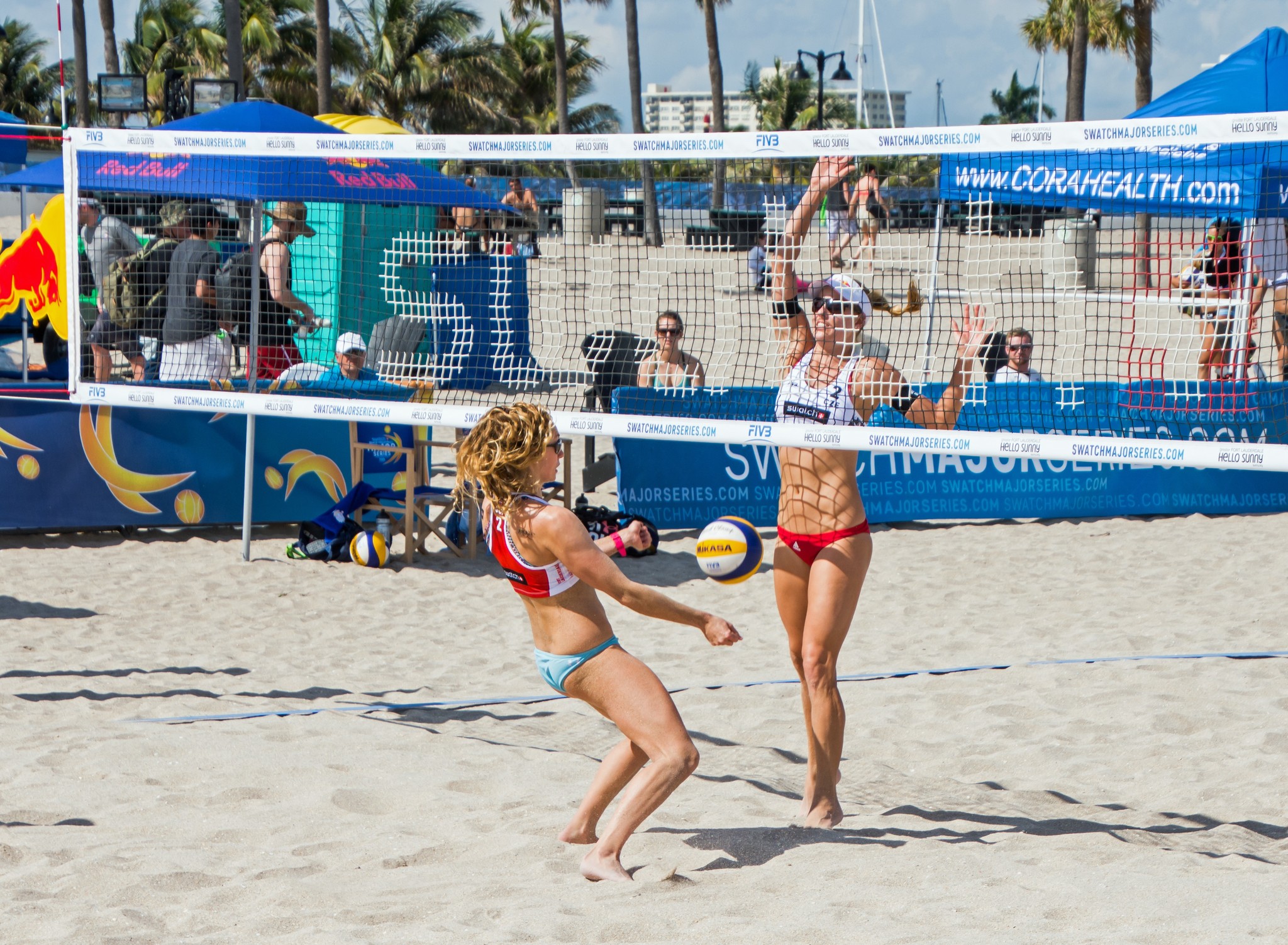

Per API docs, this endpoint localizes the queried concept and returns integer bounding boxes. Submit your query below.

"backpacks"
[215,252,251,325]
[99,236,181,328]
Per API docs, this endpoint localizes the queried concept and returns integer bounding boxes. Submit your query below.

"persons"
[748,231,815,293]
[637,311,705,389]
[846,162,890,272]
[435,176,542,259]
[826,175,858,267]
[76,190,145,383]
[992,327,1047,383]
[1171,214,1288,383]
[132,200,193,382]
[158,202,245,381]
[233,200,317,378]
[772,152,1000,838]
[317,330,397,381]
[457,403,743,884]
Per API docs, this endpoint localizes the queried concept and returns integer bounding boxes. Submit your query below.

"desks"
[708,209,770,252]
[497,198,562,229]
[604,199,644,238]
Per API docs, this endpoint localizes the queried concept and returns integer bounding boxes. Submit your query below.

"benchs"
[684,223,722,251]
[436,230,485,252]
[548,213,666,238]
[756,227,784,252]
[954,213,1015,237]
[1045,212,1101,229]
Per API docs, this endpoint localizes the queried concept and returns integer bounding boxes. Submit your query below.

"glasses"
[1207,235,1222,243]
[546,437,562,454]
[1008,343,1031,351]
[812,297,854,314]
[510,182,519,187]
[656,327,681,337]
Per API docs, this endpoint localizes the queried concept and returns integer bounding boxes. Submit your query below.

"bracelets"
[771,297,803,320]
[892,386,922,416]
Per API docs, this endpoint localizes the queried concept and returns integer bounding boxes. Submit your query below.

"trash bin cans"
[625,187,645,230]
[1042,218,1096,289]
[560,187,606,246]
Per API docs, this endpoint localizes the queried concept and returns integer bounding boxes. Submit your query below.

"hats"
[336,332,366,353]
[809,276,873,324]
[263,202,316,238]
[155,200,189,226]
[186,202,229,221]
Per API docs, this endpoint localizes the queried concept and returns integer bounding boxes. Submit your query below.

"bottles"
[215,329,225,340]
[298,316,311,337]
[575,493,588,507]
[376,509,391,548]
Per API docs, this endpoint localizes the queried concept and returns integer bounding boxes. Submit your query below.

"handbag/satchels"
[571,506,659,558]
[866,177,885,218]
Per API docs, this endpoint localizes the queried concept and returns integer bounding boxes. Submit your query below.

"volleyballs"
[349,530,390,568]
[697,517,763,586]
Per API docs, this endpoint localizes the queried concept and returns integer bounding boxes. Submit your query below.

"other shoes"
[832,253,846,268]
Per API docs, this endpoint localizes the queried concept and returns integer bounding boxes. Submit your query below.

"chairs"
[455,428,572,510]
[978,332,1008,381]
[580,330,660,494]
[349,421,476,564]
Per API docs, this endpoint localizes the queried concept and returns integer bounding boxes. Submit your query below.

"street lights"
[789,49,854,130]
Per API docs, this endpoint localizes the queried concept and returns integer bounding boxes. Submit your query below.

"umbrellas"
[0,97,527,562]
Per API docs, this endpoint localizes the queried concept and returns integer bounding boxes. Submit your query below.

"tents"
[921,25,1288,388]
[0,106,32,383]
